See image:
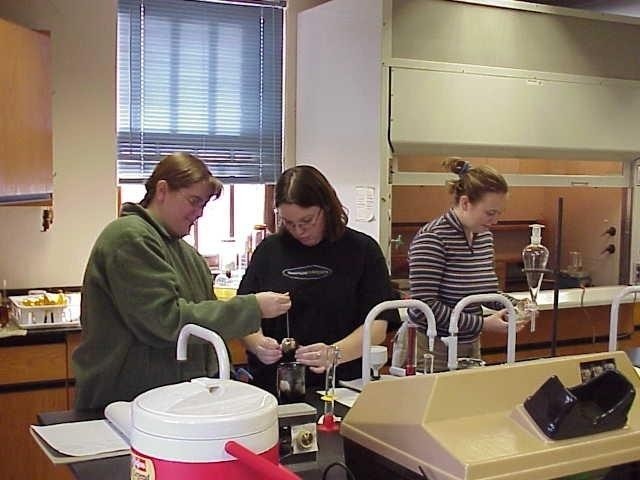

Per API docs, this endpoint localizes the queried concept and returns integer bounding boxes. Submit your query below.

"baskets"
[8,289,71,327]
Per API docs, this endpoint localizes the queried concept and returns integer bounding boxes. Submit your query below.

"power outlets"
[608,243,616,256]
[633,262,640,281]
[607,225,617,242]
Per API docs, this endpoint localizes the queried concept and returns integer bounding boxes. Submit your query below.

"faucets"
[448,292,518,366]
[362,298,438,388]
[173,320,234,381]
[609,284,640,350]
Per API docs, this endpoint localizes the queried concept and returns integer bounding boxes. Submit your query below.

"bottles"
[423,353,434,373]
[325,346,335,416]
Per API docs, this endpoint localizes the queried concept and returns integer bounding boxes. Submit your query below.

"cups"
[277,361,306,400]
[569,251,583,271]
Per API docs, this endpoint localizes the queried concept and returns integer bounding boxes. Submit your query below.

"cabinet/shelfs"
[0,331,81,480]
[0,18,51,205]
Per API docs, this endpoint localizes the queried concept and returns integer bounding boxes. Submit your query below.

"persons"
[389,156,538,372]
[234,166,401,386]
[71,152,291,411]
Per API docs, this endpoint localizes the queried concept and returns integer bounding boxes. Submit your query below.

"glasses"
[184,196,207,208]
[281,209,320,231]
